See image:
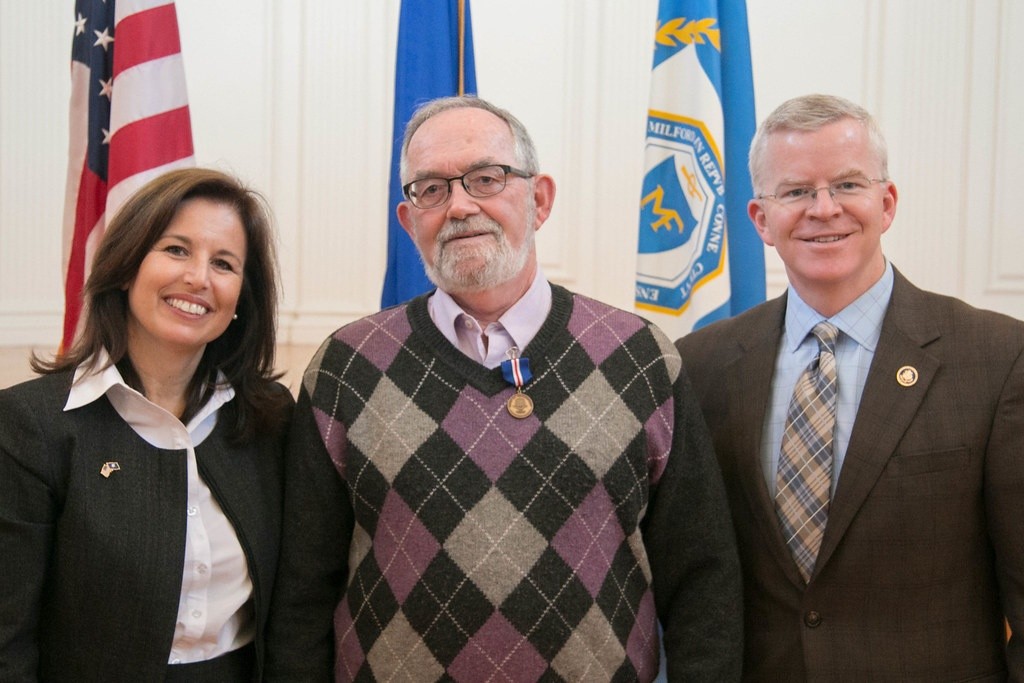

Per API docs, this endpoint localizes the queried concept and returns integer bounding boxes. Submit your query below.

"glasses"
[758,176,887,209]
[403,164,533,209]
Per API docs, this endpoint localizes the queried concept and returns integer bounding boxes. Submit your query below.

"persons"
[297,96,742,683]
[0,166,295,683]
[676,93,1024,682]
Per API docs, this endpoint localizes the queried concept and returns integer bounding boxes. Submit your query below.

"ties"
[775,321,839,584]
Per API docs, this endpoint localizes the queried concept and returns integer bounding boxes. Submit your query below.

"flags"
[57,0,196,366]
[636,1,780,338]
[379,0,479,312]
